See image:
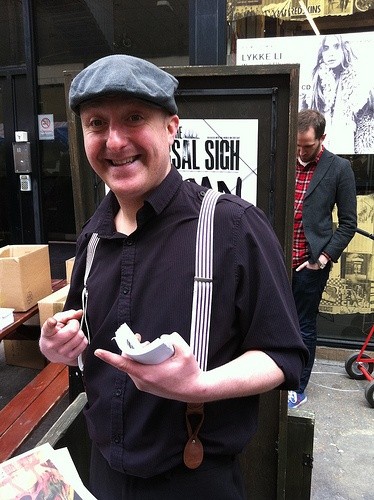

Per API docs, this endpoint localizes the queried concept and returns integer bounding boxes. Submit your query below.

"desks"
[0,278,66,342]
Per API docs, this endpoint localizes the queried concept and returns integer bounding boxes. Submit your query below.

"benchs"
[0,363,69,465]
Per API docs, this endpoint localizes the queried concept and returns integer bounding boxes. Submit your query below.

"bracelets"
[321,252,331,260]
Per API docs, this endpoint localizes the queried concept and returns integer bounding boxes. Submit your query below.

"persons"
[38,55,311,500]
[298,35,374,154]
[288,110,358,408]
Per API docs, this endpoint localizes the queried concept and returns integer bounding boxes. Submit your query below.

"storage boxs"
[0,242,75,370]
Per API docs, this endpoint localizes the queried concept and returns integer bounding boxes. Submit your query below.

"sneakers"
[288,391,307,410]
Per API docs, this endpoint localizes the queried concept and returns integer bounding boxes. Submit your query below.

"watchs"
[316,260,325,269]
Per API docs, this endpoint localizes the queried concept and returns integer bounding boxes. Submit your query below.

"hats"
[69,55,179,118]
